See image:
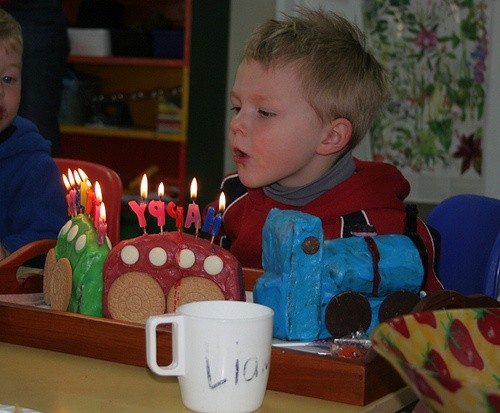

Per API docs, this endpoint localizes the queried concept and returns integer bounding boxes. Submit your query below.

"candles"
[184,176,202,238]
[127,174,149,235]
[165,201,183,235]
[147,182,166,234]
[202,190,227,243]
[61,167,108,246]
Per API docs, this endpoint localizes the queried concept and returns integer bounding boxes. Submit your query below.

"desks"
[0,339,423,413]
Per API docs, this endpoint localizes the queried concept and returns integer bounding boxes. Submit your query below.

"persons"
[202,10,445,298]
[0,9,67,269]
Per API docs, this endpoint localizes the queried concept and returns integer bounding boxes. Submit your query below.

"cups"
[144,299,274,412]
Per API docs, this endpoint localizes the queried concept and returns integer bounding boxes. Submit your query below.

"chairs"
[423,195,500,302]
[0,159,123,298]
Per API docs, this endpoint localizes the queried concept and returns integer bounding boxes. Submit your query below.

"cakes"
[42,212,243,332]
[254,208,428,343]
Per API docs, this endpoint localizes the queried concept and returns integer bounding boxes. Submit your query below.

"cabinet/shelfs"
[54,0,230,234]
